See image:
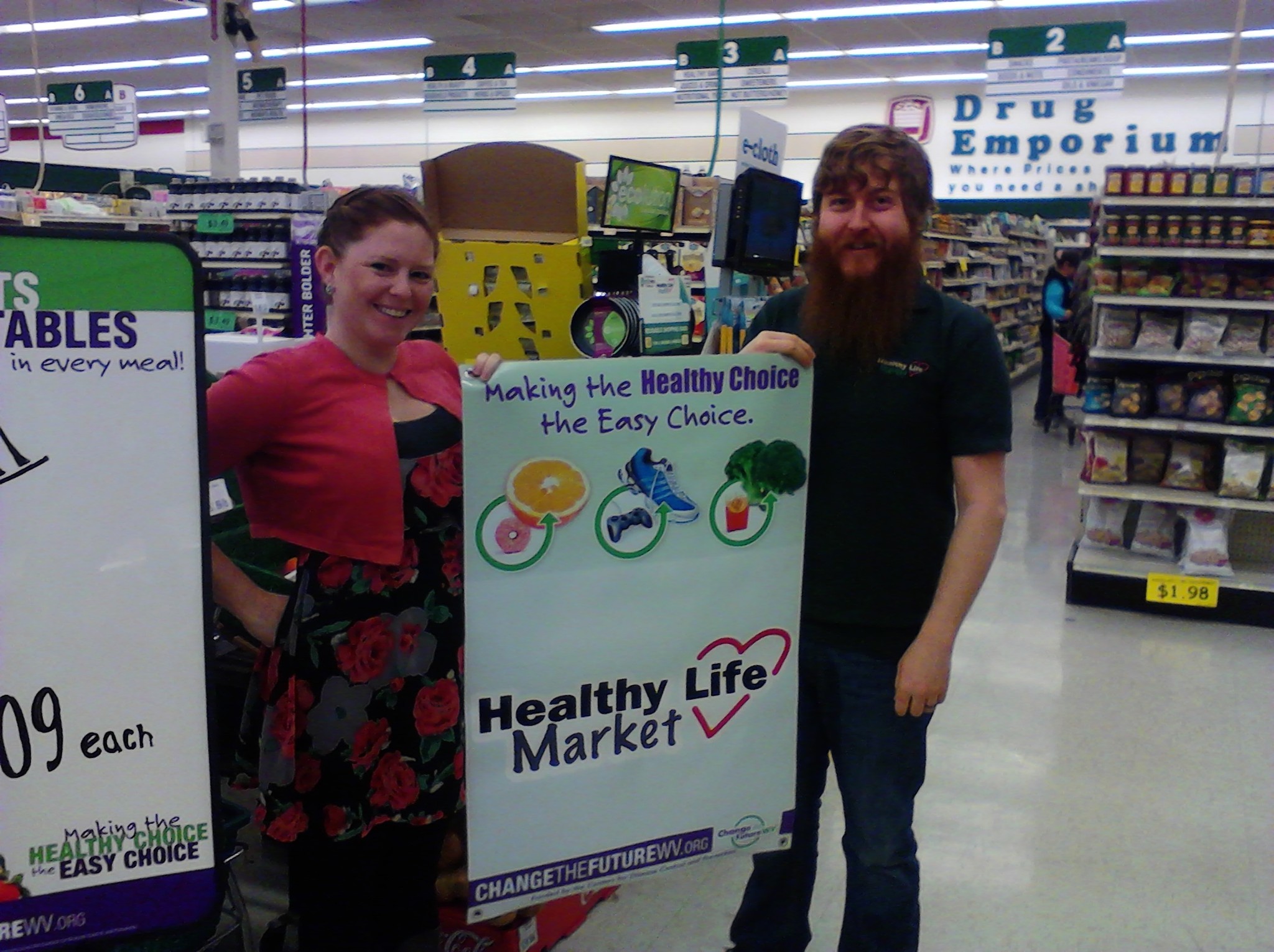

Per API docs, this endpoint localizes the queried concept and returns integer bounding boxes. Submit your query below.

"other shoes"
[1034,415,1059,428]
[1053,413,1075,429]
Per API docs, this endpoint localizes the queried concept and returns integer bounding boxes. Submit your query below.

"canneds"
[1103,163,1274,249]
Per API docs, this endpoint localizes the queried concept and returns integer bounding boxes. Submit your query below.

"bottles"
[1091,163,1274,252]
[167,176,307,336]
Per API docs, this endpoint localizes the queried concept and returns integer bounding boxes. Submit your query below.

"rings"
[925,703,936,708]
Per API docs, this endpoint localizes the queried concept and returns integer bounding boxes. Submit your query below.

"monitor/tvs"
[726,167,803,278]
[600,154,681,233]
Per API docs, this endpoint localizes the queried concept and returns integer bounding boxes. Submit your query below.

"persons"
[207,185,503,952]
[1031,249,1082,429]
[727,125,1012,952]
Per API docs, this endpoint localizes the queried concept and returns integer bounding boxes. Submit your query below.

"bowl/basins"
[569,296,640,359]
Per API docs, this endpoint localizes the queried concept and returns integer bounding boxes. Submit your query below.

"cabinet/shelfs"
[910,193,1274,633]
[0,158,817,355]
